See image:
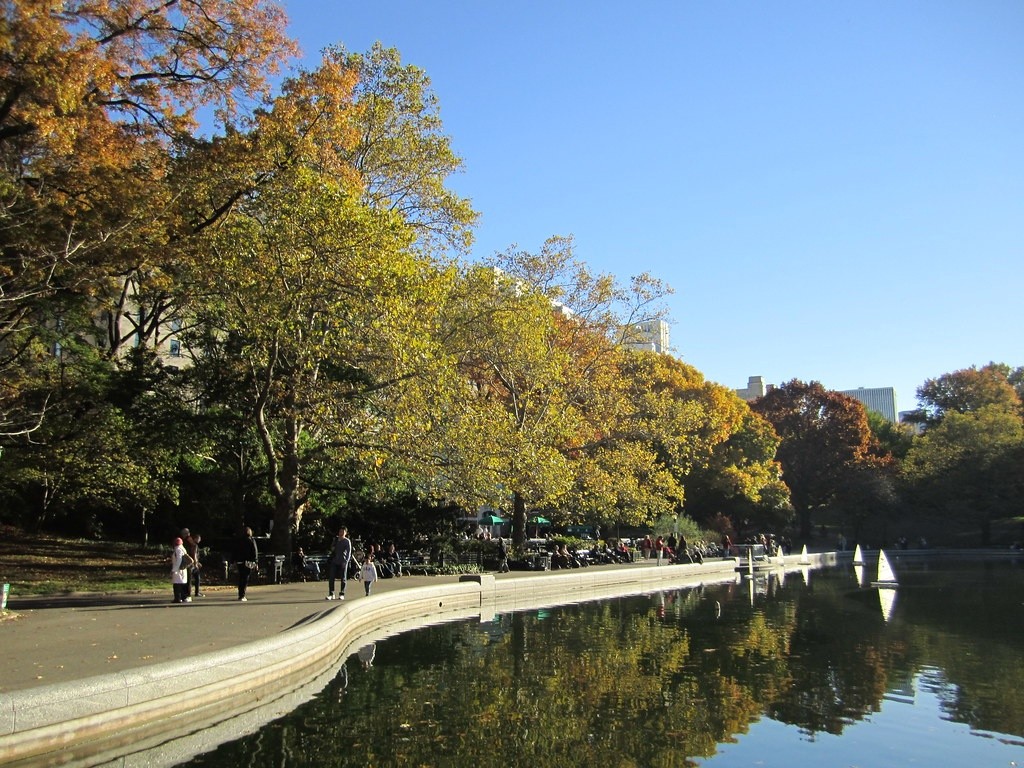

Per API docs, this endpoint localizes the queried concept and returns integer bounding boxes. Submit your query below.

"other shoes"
[171,599,182,603]
[326,595,335,600]
[338,596,344,600]
[497,571,503,573]
[504,570,510,573]
[238,597,248,601]
[185,597,192,602]
[195,593,206,597]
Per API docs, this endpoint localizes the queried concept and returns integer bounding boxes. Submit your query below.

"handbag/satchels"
[175,548,194,571]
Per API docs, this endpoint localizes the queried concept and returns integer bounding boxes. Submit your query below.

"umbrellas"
[527,515,551,539]
[478,515,504,532]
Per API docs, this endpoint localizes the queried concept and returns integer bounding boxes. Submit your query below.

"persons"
[326,526,352,601]
[466,524,492,541]
[553,544,573,569]
[544,531,554,541]
[497,537,510,573]
[830,533,929,551]
[591,542,632,565]
[365,541,403,578]
[236,526,259,601]
[295,546,321,581]
[171,527,206,603]
[359,554,378,596]
[744,533,792,557]
[643,532,733,566]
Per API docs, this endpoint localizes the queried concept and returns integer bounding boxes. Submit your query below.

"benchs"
[298,557,432,583]
[549,548,624,570]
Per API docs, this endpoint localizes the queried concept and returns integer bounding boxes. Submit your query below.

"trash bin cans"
[218,560,228,580]
[264,554,285,584]
